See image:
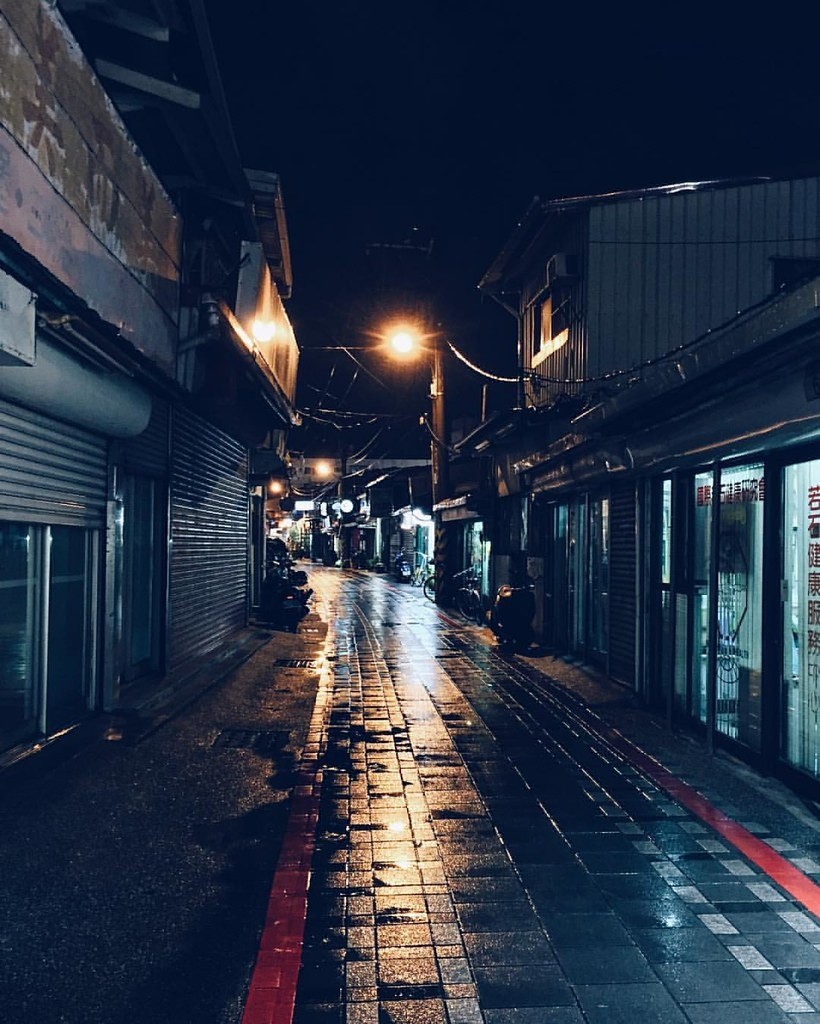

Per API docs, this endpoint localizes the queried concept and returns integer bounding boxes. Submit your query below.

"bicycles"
[422,558,457,603]
[411,551,429,587]
[453,567,484,628]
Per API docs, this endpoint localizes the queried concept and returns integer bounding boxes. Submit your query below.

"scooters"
[394,546,412,584]
[264,559,311,634]
[484,567,539,650]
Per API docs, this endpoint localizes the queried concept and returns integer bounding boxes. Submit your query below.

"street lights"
[388,320,451,603]
